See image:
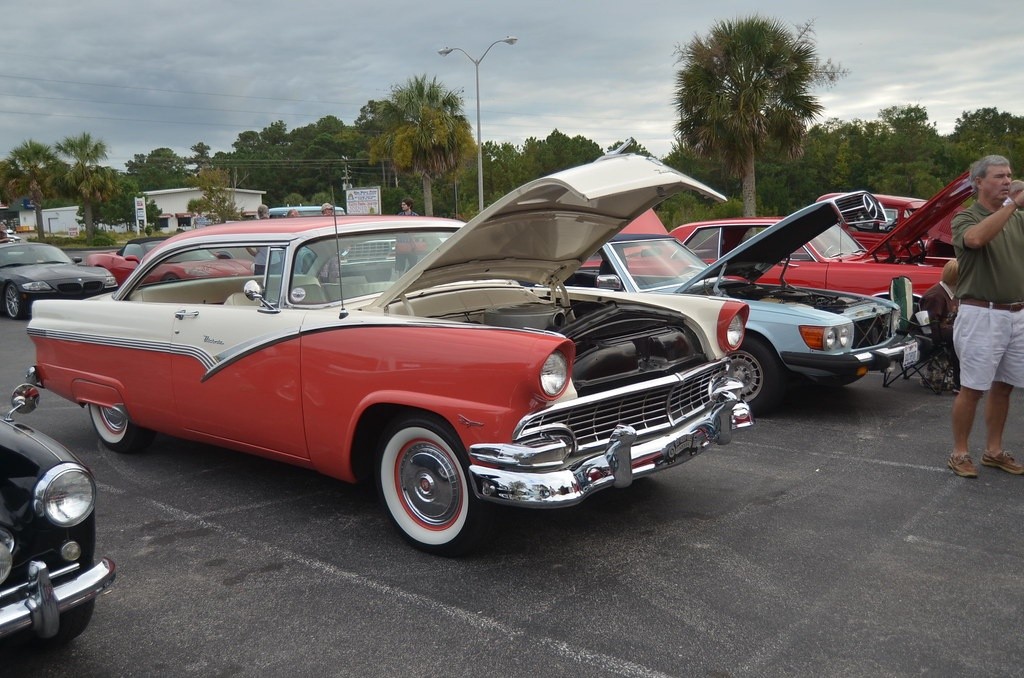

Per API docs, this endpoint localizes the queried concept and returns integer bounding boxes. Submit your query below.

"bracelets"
[1013,199,1021,208]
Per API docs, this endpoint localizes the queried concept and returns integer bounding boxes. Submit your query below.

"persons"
[947,154,1024,477]
[246,204,282,276]
[396,197,421,279]
[316,202,341,302]
[1001,180,1024,211]
[906,260,984,398]
[286,209,313,275]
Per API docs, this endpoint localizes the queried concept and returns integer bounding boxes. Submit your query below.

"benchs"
[132,272,322,305]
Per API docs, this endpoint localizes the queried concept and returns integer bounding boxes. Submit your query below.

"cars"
[815,192,967,256]
[211,206,397,282]
[0,382,116,652]
[584,168,975,319]
[26,152,755,555]
[519,190,921,408]
[0,221,21,243]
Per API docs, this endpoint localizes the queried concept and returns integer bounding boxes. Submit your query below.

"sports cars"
[85,236,255,287]
[0,242,120,321]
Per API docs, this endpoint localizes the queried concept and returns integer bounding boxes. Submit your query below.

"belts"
[961,298,1024,313]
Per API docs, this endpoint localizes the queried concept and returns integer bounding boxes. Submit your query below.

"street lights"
[438,36,517,211]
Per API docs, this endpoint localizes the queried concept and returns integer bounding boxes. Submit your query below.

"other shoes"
[952,386,984,397]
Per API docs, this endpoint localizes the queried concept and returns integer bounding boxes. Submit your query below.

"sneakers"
[948,453,977,477]
[981,449,1024,474]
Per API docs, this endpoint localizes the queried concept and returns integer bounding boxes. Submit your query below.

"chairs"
[222,285,327,307]
[882,276,959,395]
[325,281,393,301]
